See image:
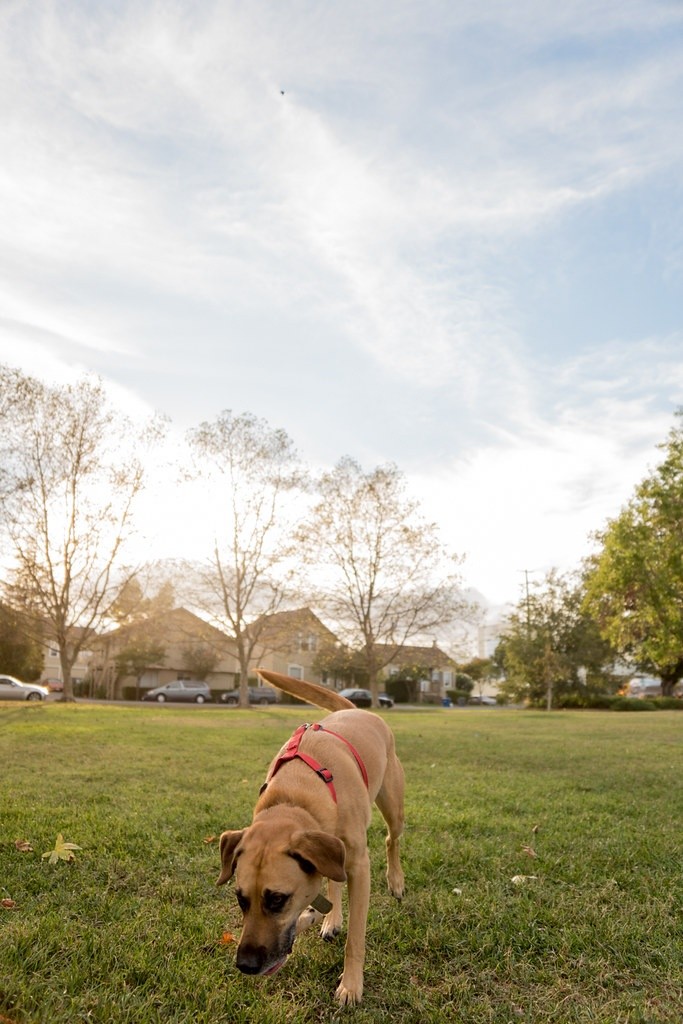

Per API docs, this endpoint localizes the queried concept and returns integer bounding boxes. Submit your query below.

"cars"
[41,678,64,692]
[470,696,498,706]
[337,688,394,708]
[0,674,50,701]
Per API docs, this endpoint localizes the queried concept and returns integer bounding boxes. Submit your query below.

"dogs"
[217,668,405,1010]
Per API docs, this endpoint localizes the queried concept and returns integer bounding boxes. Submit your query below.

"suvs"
[220,686,275,705]
[143,679,212,704]
